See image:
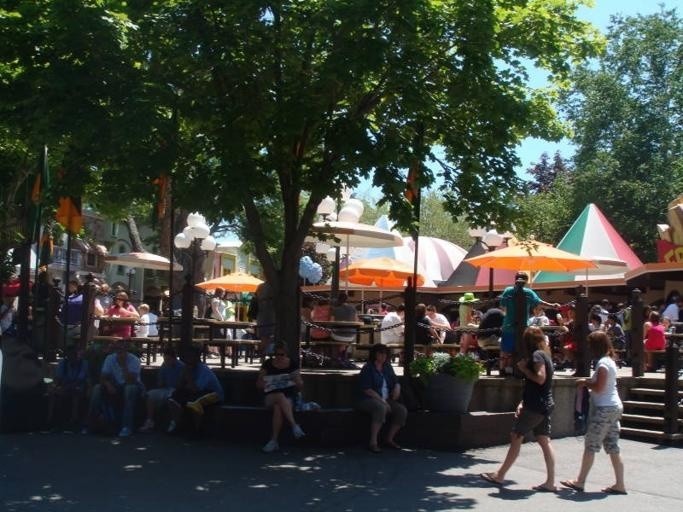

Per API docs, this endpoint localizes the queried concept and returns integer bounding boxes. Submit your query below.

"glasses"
[276,352,287,356]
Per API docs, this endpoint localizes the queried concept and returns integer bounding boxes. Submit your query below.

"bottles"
[556,313,564,323]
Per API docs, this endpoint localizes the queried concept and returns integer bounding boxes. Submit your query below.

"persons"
[254,339,304,453]
[559,330,628,495]
[301,290,506,376]
[499,271,556,377]
[527,291,682,372]
[1,262,255,449]
[354,343,408,453]
[479,324,557,492]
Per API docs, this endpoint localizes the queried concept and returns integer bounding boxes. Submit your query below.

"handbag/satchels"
[394,383,423,412]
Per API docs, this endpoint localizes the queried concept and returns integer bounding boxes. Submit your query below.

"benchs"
[411,304,683,364]
[0,318,264,367]
[300,314,404,370]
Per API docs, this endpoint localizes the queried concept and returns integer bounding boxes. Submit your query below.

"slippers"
[601,487,627,494]
[480,474,504,484]
[532,485,557,492]
[560,480,584,492]
[385,440,401,449]
[367,444,381,451]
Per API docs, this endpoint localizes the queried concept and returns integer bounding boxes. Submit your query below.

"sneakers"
[169,432,204,447]
[40,418,177,437]
[292,425,306,439]
[500,368,514,376]
[644,365,656,371]
[263,440,278,453]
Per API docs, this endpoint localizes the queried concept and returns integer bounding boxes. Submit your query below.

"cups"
[670,327,676,334]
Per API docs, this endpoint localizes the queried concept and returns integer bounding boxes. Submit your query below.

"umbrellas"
[569,255,628,297]
[463,235,601,288]
[340,256,424,313]
[194,271,265,302]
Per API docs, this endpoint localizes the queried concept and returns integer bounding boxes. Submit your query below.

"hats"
[472,311,483,319]
[515,274,528,284]
[459,292,480,303]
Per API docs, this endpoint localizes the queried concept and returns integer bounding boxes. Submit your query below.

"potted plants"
[410,355,484,412]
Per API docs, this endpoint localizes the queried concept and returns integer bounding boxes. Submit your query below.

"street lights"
[315,184,362,302]
[172,211,216,317]
[124,266,136,289]
[467,224,516,298]
